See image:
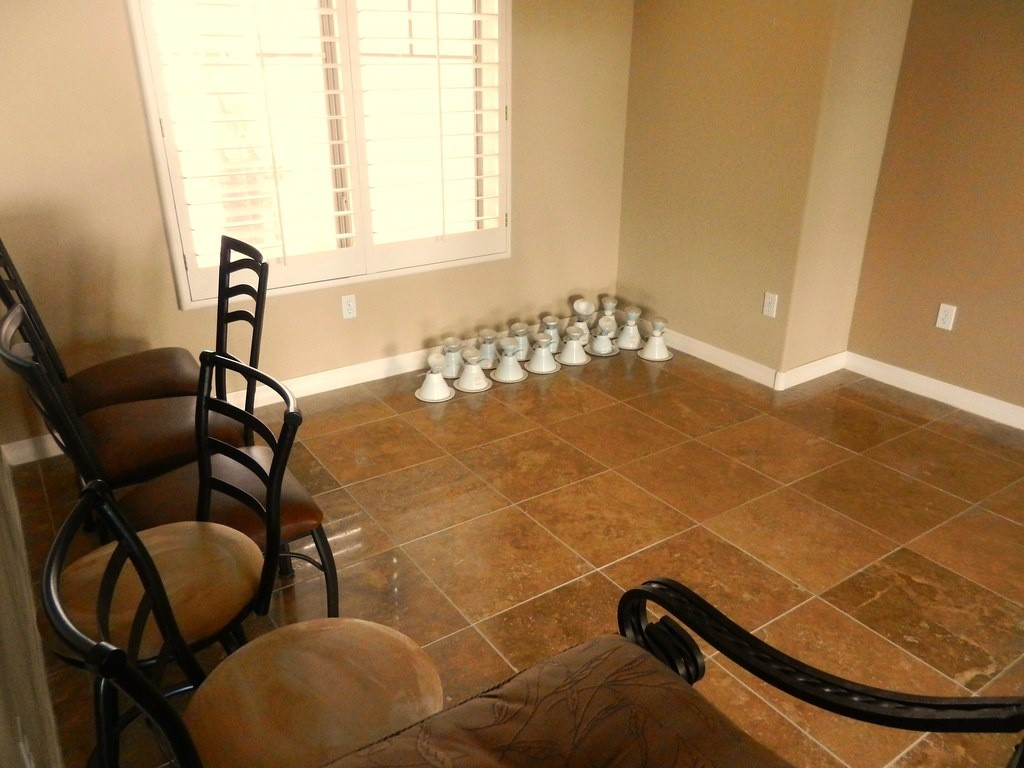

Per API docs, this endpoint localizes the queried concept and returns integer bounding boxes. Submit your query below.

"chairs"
[324,577,1023,768]
[75,234,270,488]
[35,349,303,768]
[41,476,446,768]
[0,237,200,411]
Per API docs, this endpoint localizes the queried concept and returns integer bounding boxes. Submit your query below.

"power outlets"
[935,302,957,331]
[763,292,778,318]
[341,294,356,320]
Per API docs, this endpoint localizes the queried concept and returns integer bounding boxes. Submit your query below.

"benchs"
[0,302,339,617]
